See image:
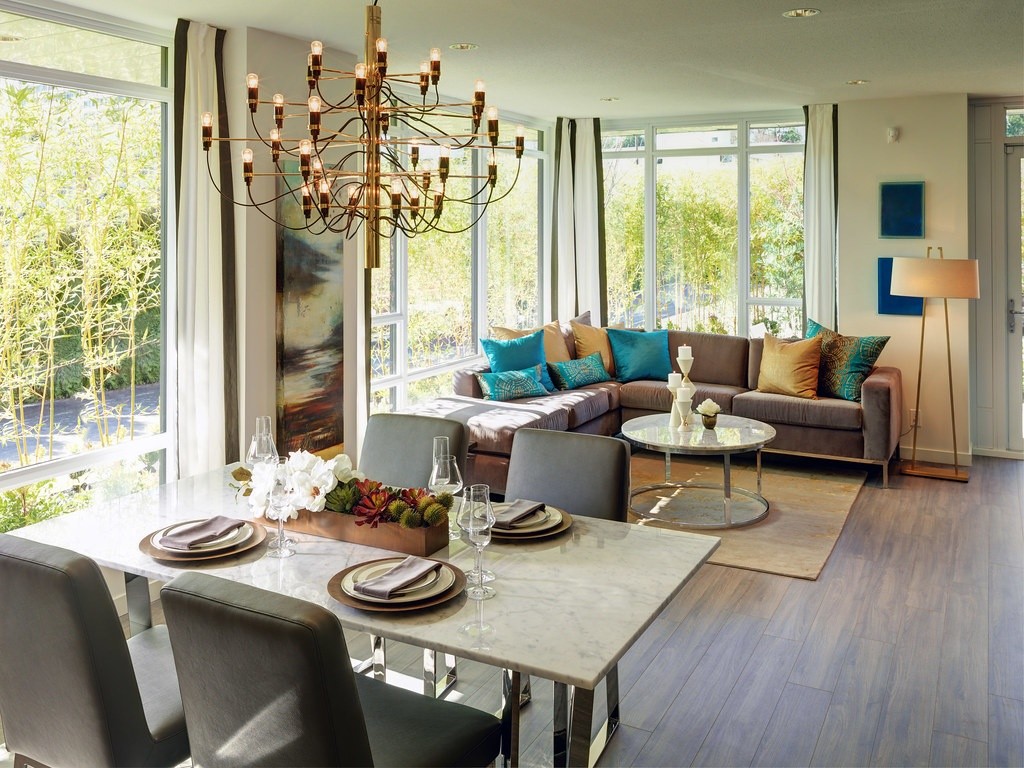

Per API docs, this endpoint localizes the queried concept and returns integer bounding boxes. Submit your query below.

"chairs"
[0,413,632,767]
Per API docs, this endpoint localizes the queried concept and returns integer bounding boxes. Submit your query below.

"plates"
[460,504,573,538]
[139,520,266,561]
[327,557,467,611]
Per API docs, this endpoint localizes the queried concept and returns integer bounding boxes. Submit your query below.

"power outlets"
[910,409,921,427]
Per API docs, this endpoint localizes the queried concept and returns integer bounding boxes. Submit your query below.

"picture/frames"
[877,181,926,239]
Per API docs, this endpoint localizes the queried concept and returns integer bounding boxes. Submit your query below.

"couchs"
[413,329,905,499]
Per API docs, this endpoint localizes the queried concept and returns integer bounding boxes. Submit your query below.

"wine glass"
[456,486,495,583]
[265,456,301,558]
[428,436,463,541]
[467,484,496,600]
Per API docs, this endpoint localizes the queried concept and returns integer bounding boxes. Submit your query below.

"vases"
[702,414,718,430]
[253,508,450,557]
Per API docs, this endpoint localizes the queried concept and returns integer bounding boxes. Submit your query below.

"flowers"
[691,397,721,416]
[229,449,455,529]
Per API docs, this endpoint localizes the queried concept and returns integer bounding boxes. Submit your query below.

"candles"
[667,370,681,387]
[677,387,691,401]
[678,343,693,358]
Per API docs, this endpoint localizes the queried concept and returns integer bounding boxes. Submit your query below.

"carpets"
[628,452,872,581]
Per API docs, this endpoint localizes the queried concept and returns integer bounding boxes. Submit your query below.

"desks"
[5,463,721,768]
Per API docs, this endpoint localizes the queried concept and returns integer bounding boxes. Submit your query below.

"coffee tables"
[621,413,777,532]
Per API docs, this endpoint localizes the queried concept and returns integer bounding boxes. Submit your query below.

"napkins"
[488,499,547,530]
[354,555,443,601]
[159,516,245,551]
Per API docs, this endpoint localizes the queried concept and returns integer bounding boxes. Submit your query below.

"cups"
[246,434,279,465]
[255,416,271,437]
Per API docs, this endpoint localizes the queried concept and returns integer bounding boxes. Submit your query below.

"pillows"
[473,310,674,402]
[756,317,891,401]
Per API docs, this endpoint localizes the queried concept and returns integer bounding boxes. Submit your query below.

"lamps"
[201,1,528,270]
[890,247,980,482]
